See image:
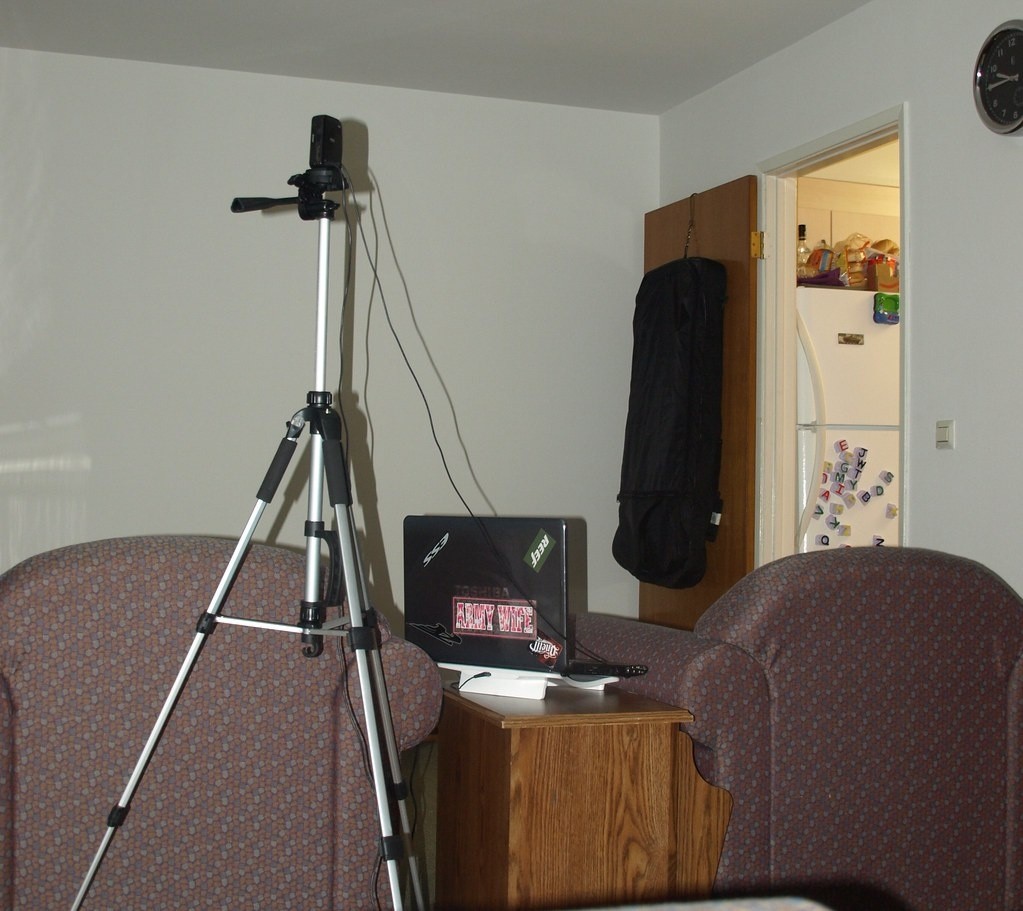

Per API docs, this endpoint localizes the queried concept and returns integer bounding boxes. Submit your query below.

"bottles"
[797,224,811,264]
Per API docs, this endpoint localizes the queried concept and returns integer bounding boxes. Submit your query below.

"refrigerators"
[795,286,899,554]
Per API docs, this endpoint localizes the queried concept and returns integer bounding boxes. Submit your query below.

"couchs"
[0,531,447,911]
[576,547,1023,911]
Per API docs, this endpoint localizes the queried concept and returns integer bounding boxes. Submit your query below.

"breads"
[794,237,900,294]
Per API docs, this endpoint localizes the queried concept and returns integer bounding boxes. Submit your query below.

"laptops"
[402,515,648,677]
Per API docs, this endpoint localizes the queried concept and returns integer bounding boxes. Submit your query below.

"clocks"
[971,19,1023,134]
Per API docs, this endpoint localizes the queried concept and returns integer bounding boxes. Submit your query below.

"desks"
[433,677,732,911]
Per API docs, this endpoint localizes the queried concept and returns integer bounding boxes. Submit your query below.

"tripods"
[71,166,430,911]
